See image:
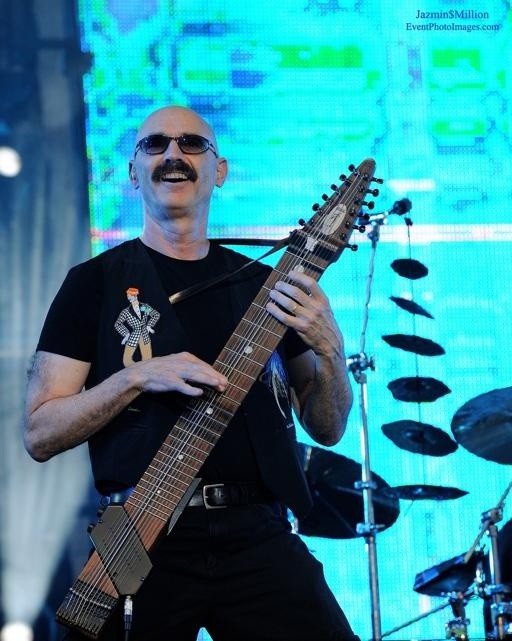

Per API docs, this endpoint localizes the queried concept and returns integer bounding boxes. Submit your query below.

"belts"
[110,482,229,509]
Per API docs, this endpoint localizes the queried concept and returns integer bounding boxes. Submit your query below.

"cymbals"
[379,421,458,456]
[292,438,397,537]
[383,334,444,356]
[391,260,428,279]
[454,387,511,465]
[389,295,434,321]
[393,486,467,500]
[389,376,451,402]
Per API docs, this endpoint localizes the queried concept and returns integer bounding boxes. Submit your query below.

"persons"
[22,104,360,639]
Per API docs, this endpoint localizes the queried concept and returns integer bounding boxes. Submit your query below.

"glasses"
[133,134,217,161]
